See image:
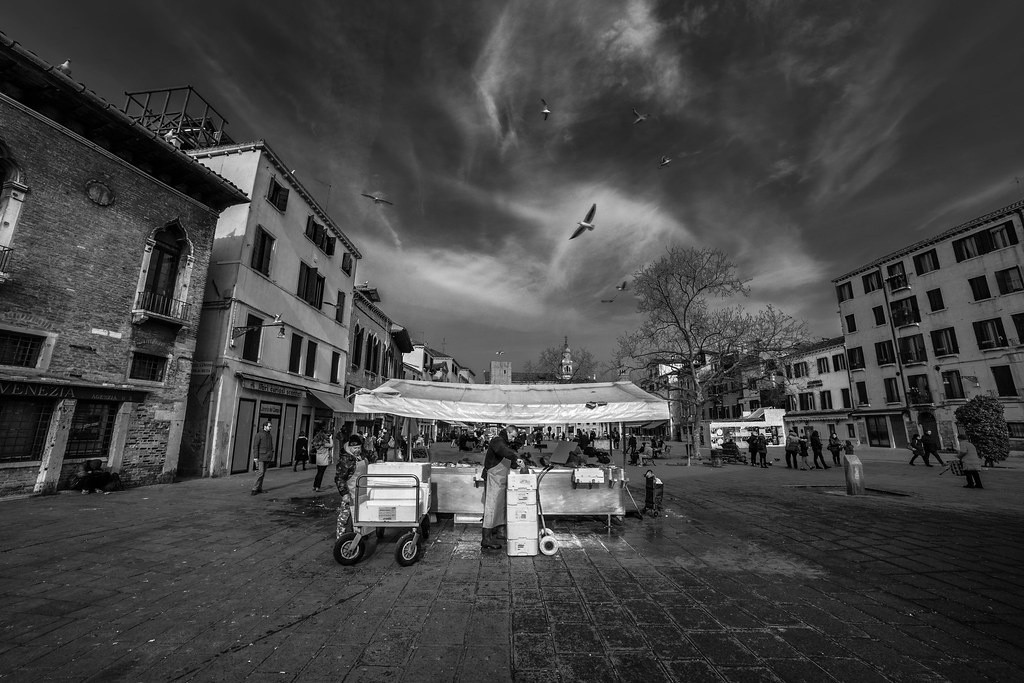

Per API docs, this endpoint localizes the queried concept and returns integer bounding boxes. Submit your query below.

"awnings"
[642,421,667,429]
[306,389,368,420]
[749,407,772,418]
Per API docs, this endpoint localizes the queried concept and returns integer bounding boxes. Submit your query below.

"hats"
[348,435,362,446]
[913,434,920,438]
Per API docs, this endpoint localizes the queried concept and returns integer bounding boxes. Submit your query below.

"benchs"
[661,445,674,459]
[643,448,657,466]
[710,450,748,465]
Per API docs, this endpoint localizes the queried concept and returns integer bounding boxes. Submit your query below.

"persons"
[293,431,309,471]
[612,427,620,449]
[785,430,853,470]
[450,428,457,448]
[574,430,596,450]
[474,428,488,451]
[518,429,565,453]
[312,430,333,492]
[957,435,984,489]
[357,427,391,462]
[459,431,474,451]
[339,425,349,449]
[251,421,274,496]
[651,436,665,458]
[626,435,646,465]
[746,432,769,468]
[482,424,525,549]
[334,435,384,539]
[909,430,949,467]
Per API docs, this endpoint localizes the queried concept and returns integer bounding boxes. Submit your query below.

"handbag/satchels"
[827,444,832,450]
[906,438,918,451]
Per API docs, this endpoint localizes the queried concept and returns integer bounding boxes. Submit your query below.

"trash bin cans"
[685,444,694,456]
[710,450,723,467]
[310,449,317,464]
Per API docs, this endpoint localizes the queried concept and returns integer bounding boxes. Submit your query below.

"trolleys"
[334,473,430,567]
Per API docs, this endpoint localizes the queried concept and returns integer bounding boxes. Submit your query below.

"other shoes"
[963,484,974,489]
[303,468,306,470]
[313,487,316,490]
[800,468,807,471]
[316,488,324,492]
[82,489,89,495]
[942,463,949,466]
[492,534,506,540]
[765,466,768,468]
[293,468,297,472]
[816,466,824,469]
[258,490,268,494]
[252,490,257,496]
[838,463,841,466]
[482,541,502,549]
[927,465,933,467]
[825,466,831,469]
[95,489,103,494]
[975,485,984,489]
[761,466,764,467]
[810,466,815,470]
[910,462,916,466]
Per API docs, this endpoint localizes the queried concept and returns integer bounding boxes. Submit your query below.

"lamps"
[942,375,981,388]
[232,323,286,340]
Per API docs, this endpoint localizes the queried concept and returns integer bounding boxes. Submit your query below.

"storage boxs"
[507,522,538,540]
[507,505,537,522]
[506,488,537,506]
[507,539,538,557]
[350,462,432,523]
[507,473,538,490]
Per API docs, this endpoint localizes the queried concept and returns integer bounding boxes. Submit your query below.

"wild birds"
[616,281,629,292]
[569,204,597,240]
[361,194,393,205]
[541,98,551,122]
[57,58,72,75]
[161,128,174,143]
[660,156,672,167]
[632,108,651,125]
